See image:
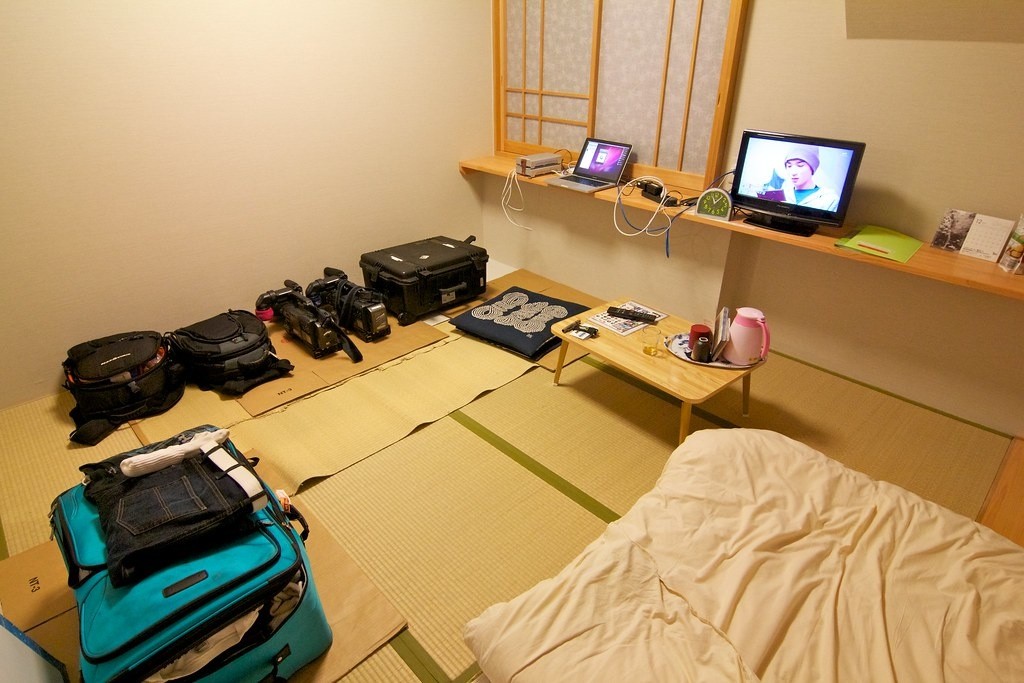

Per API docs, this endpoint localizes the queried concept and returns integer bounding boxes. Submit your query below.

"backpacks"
[163,309,296,398]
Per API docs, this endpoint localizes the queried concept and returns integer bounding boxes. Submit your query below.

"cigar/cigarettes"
[562,320,581,333]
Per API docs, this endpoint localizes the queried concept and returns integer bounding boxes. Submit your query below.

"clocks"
[694,187,733,222]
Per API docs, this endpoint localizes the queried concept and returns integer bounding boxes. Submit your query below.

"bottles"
[691,337,711,362]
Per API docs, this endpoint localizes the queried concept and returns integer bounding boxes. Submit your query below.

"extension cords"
[642,190,677,206]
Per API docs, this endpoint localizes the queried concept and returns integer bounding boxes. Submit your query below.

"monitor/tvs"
[730,129,866,237]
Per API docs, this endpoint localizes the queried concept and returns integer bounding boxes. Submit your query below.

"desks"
[458,155,1024,302]
[551,296,766,445]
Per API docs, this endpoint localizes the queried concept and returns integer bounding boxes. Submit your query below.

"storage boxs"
[358,235,490,328]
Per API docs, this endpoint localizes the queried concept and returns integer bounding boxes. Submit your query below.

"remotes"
[607,306,656,324]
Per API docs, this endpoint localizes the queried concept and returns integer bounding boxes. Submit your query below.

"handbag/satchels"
[63,330,188,446]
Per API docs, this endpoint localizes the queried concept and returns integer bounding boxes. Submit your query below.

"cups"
[688,323,710,348]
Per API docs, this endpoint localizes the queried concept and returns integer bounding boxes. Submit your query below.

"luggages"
[46,422,334,678]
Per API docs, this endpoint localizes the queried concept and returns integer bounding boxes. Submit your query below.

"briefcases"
[359,234,491,328]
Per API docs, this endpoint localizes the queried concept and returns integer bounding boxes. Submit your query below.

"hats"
[785,143,820,174]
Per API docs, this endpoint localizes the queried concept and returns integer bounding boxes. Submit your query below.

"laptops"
[543,137,633,193]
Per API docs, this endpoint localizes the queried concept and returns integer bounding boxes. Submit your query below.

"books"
[711,307,730,361]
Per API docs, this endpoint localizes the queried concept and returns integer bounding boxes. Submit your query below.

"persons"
[779,146,840,212]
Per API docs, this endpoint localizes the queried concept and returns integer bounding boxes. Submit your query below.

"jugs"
[723,308,770,365]
